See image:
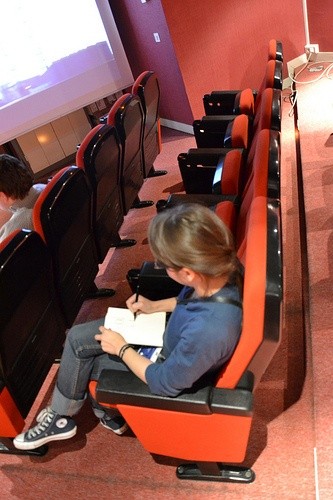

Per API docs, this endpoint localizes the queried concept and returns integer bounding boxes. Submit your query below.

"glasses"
[154,257,172,270]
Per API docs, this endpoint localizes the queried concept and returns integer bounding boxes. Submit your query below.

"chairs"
[0,39,284,484]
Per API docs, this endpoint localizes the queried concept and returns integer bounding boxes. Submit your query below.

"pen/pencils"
[134,286,141,319]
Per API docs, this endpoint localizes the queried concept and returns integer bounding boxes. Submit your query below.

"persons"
[0,154,48,245]
[14,203,245,450]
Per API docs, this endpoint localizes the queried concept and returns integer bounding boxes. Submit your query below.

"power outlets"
[304,44,319,54]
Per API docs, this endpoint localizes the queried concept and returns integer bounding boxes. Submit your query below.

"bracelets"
[119,345,131,358]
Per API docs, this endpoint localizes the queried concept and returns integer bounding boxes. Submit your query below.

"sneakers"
[13,406,77,450]
[98,412,128,435]
